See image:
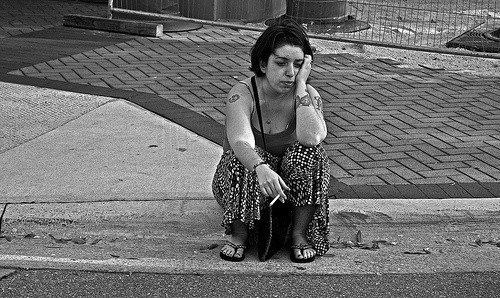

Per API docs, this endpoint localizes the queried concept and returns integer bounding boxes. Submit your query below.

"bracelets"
[252,162,267,175]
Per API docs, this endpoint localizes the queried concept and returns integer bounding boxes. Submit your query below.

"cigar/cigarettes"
[269,189,285,206]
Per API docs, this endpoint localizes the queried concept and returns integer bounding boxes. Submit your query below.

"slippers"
[290,243,315,263]
[220,241,246,262]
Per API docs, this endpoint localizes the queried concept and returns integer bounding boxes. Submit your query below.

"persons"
[211,20,331,263]
